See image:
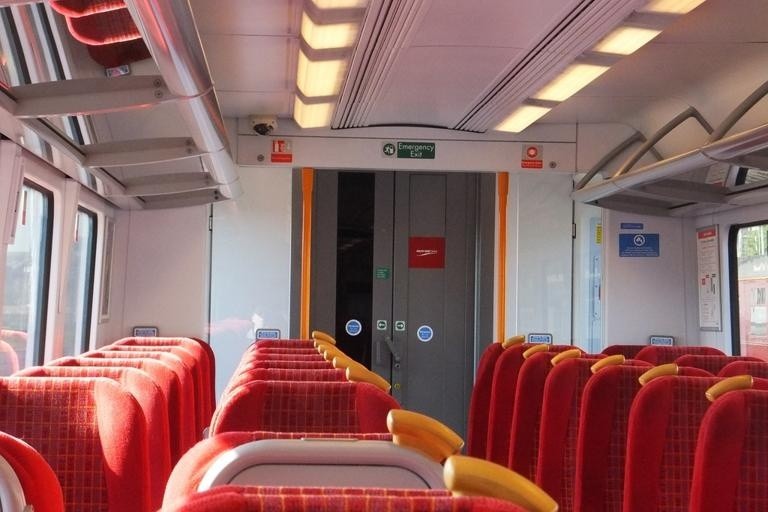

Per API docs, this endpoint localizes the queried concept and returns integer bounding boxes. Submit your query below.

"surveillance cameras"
[250,115,277,139]
